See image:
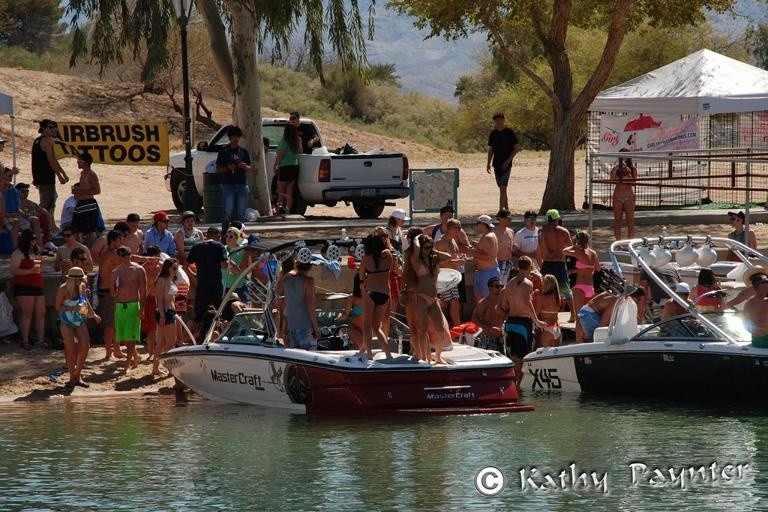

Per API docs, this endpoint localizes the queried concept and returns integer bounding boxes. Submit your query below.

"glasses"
[48,125,56,129]
[172,267,178,270]
[528,217,535,220]
[77,258,87,261]
[491,284,505,288]
[63,234,71,238]
[22,190,29,192]
[118,252,131,256]
[423,244,434,250]
[290,116,297,120]
[32,237,37,240]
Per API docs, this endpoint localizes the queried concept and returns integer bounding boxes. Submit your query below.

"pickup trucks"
[163,119,408,219]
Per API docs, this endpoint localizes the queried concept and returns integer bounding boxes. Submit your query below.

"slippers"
[22,341,48,350]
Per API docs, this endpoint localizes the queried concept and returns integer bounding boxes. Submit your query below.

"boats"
[519,236,768,411]
[155,237,534,415]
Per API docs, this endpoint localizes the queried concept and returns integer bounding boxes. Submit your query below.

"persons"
[0,112,768,388]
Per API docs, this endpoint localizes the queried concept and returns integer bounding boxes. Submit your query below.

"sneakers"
[45,242,58,252]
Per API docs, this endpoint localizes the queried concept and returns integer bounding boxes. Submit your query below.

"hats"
[65,266,88,279]
[439,206,454,214]
[493,112,505,119]
[38,119,57,132]
[524,210,537,216]
[15,182,30,191]
[128,211,241,236]
[675,282,691,292]
[727,211,745,221]
[0,136,9,143]
[391,209,411,221]
[546,209,560,220]
[742,266,768,287]
[477,214,495,228]
[76,152,93,164]
